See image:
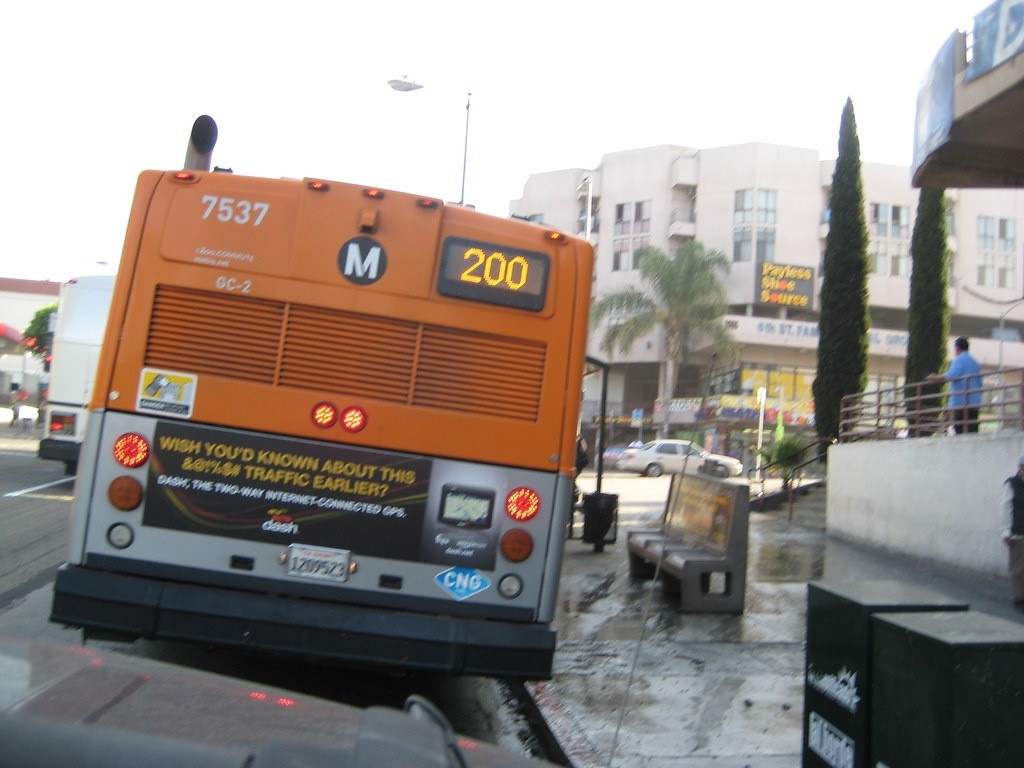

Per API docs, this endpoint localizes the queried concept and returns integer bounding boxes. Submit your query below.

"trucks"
[37,274,118,478]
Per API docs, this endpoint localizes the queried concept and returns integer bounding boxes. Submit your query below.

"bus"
[48,111,596,708]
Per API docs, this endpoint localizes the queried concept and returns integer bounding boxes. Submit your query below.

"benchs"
[626,473,750,614]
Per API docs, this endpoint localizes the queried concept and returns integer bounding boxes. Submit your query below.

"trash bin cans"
[801,581,1023,767]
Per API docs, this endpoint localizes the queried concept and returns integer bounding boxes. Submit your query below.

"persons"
[1001,455,1023,616]
[924,337,983,433]
[9,400,20,427]
[628,438,642,447]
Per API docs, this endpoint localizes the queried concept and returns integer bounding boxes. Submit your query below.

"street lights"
[385,76,473,205]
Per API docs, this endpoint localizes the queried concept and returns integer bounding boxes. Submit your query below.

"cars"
[615,438,744,479]
